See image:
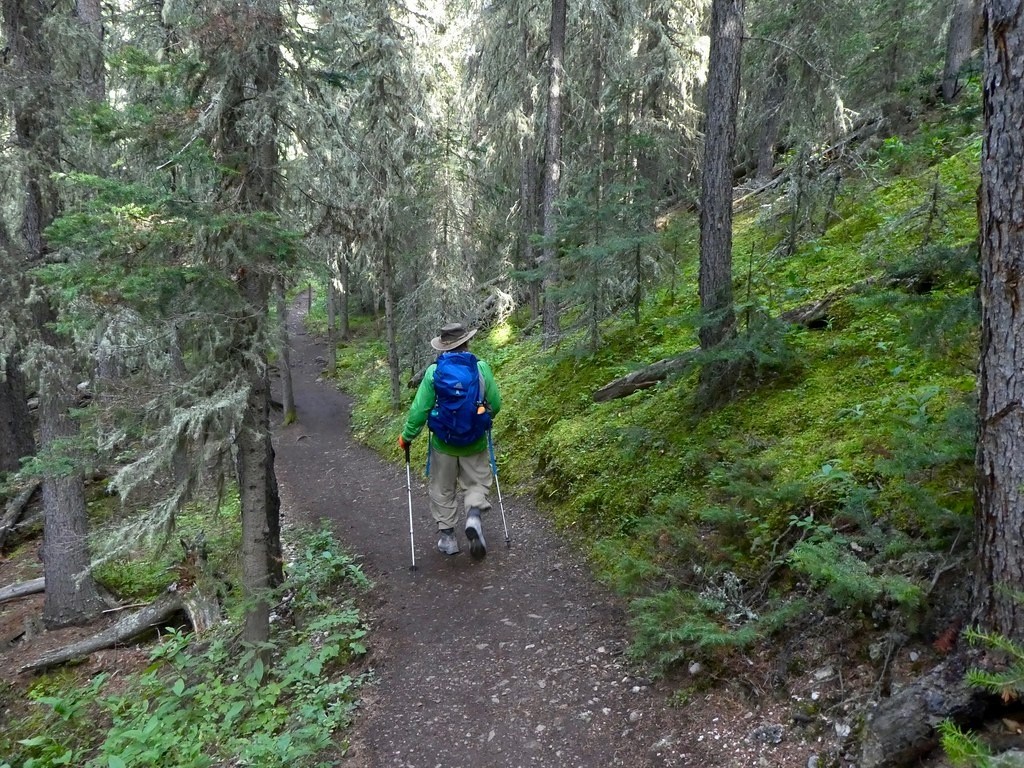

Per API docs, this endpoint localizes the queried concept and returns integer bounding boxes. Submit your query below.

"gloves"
[399,435,412,449]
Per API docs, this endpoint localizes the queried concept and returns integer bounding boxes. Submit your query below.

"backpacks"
[427,352,492,446]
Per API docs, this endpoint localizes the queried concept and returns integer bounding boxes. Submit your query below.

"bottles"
[477,404,485,414]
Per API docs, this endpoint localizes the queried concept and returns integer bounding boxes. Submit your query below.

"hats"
[430,322,477,350]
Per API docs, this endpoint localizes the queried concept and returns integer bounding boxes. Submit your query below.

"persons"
[398,323,501,559]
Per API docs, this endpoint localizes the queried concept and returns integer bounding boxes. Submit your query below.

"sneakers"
[464,517,486,559]
[437,532,459,554]
